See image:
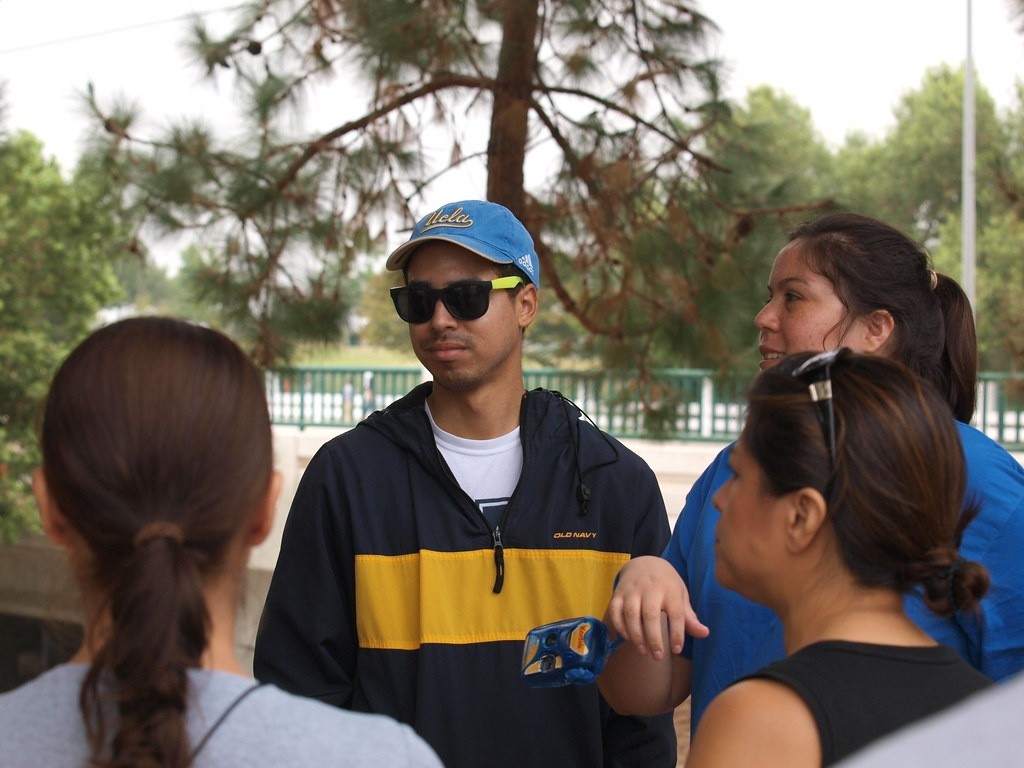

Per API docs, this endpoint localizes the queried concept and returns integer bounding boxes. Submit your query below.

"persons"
[521,213,1024,750]
[2,315,447,768]
[253,198,679,768]
[682,347,1002,768]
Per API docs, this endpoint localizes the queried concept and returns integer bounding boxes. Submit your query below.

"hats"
[386,199,543,289]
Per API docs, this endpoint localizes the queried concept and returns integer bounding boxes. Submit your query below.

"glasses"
[389,276,527,325]
[791,347,858,473]
